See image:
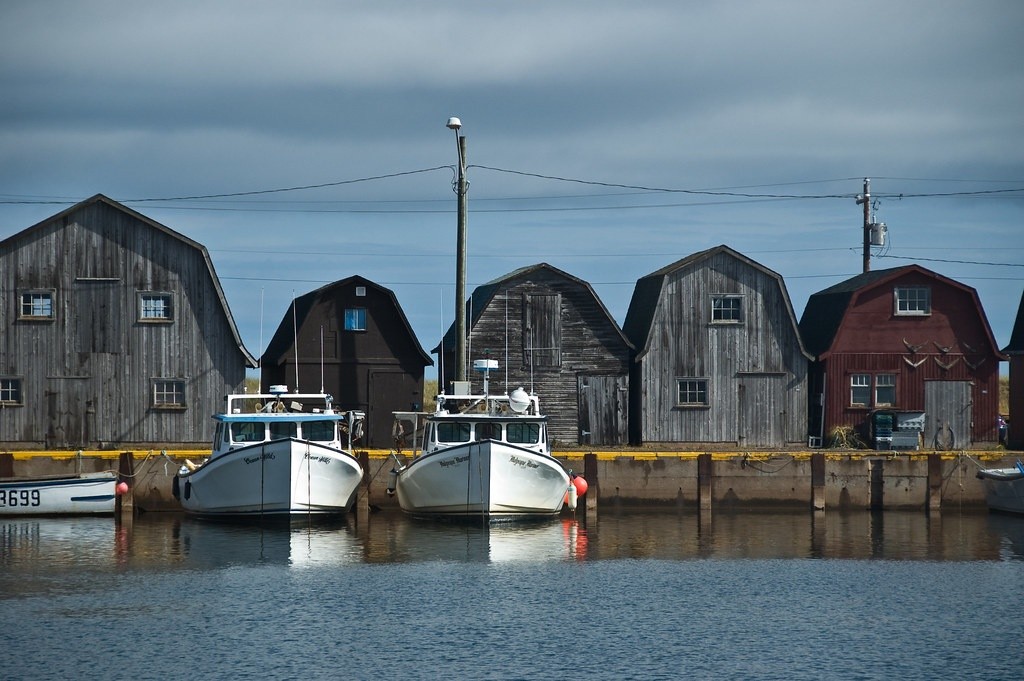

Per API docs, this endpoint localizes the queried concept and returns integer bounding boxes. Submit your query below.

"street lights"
[445,116,468,382]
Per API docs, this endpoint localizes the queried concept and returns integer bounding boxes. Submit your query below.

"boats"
[976,461,1024,517]
[391,287,569,524]
[178,287,362,528]
[0,474,117,519]
